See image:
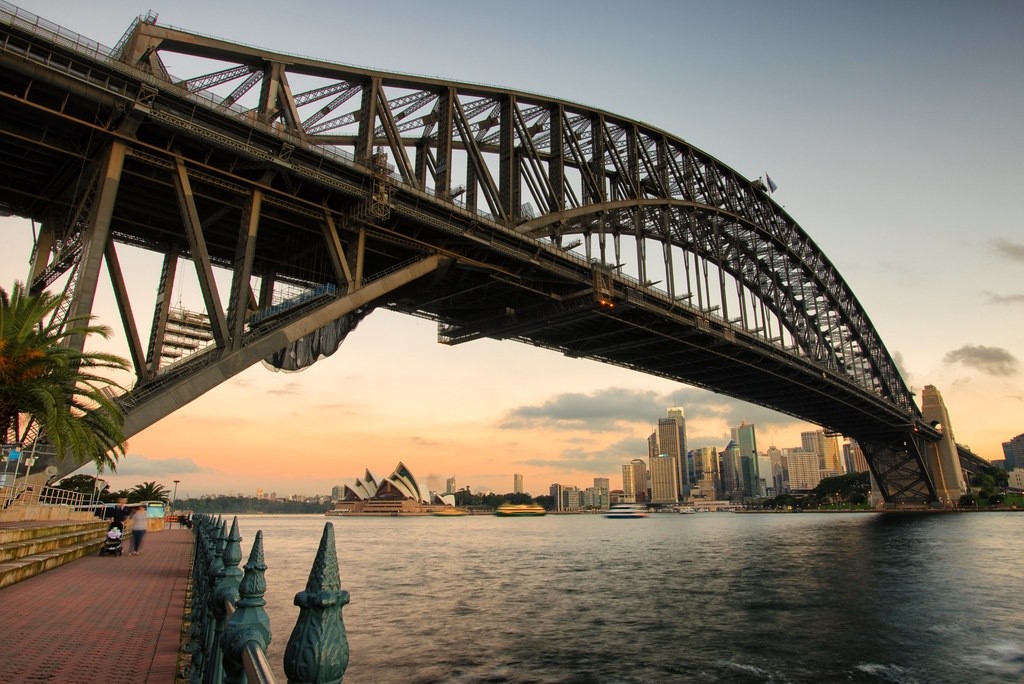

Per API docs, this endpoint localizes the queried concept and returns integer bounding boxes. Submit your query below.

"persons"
[128,505,149,555]
[114,498,131,524]
[178,511,194,529]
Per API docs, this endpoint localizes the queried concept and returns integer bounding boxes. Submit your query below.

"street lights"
[169,480,181,529]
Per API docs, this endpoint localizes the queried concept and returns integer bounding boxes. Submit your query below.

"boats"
[680,508,696,514]
[604,504,648,517]
[495,505,546,517]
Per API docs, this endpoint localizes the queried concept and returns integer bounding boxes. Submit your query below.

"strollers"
[98,521,125,559]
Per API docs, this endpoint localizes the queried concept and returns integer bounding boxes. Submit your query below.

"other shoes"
[131,551,140,556]
